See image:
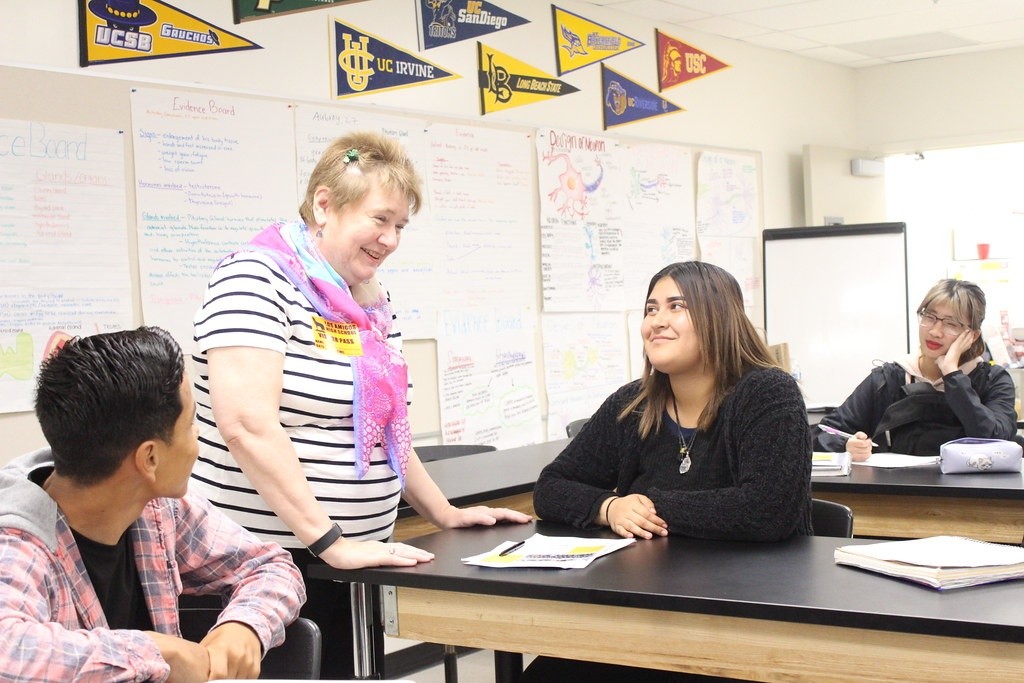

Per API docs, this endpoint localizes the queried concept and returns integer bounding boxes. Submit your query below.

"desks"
[305,522,1023,683]
[393,437,574,543]
[811,465,1024,546]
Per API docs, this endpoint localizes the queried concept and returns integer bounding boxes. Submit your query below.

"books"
[812,452,851,476]
[833,535,1024,590]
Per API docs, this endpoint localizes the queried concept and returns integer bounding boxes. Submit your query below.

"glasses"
[916,310,972,335]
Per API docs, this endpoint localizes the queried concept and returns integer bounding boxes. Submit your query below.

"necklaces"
[672,396,697,475]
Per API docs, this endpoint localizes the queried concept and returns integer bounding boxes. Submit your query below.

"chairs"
[566,418,592,438]
[811,499,853,538]
[179,609,321,680]
[411,445,498,463]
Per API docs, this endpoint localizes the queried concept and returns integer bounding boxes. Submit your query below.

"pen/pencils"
[499,540,526,556]
[818,425,879,447]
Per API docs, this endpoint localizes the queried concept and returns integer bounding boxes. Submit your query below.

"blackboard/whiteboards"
[762,222,910,412]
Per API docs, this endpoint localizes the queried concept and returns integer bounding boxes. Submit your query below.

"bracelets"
[606,498,617,523]
[306,524,343,557]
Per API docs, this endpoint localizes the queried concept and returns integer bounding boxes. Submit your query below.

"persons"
[0,326,306,683]
[187,129,534,682]
[519,262,813,682]
[814,279,1018,463]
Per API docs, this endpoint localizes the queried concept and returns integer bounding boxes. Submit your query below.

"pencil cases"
[936,437,1023,474]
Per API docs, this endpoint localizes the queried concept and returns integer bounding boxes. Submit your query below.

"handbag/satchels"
[939,438,1022,473]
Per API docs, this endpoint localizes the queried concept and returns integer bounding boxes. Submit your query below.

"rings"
[390,547,395,553]
